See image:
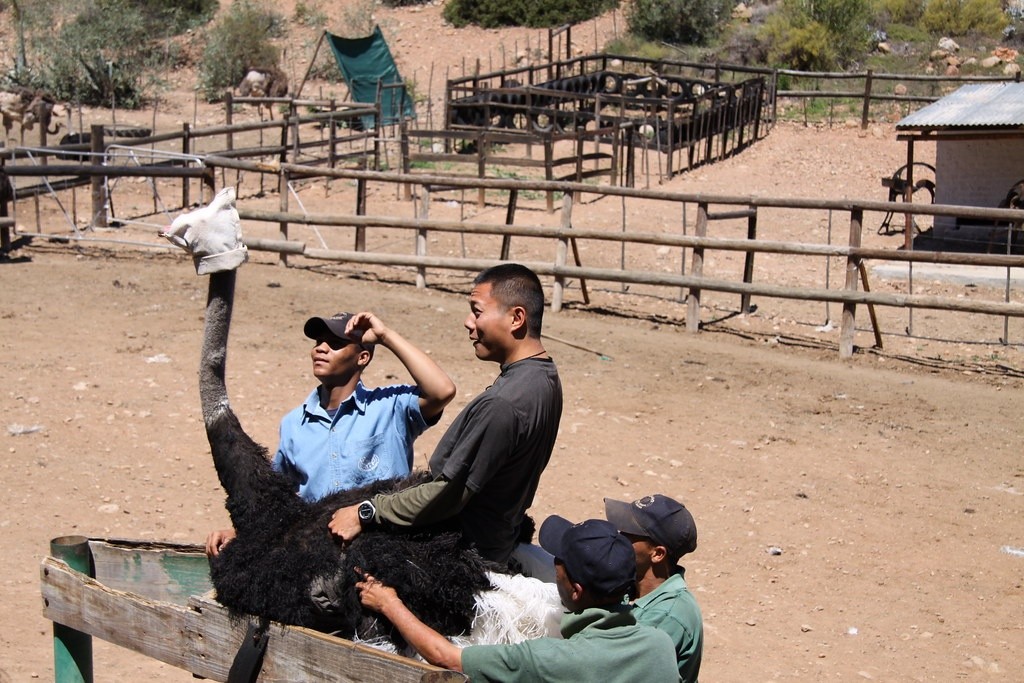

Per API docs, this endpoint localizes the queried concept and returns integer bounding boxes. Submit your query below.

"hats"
[603,494,697,558]
[304,312,374,342]
[538,516,637,601]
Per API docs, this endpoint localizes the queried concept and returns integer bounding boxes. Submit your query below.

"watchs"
[358,499,376,531]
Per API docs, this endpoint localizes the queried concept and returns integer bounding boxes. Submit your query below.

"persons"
[329,264,564,541]
[354,515,682,683]
[206,311,457,557]
[603,494,703,683]
